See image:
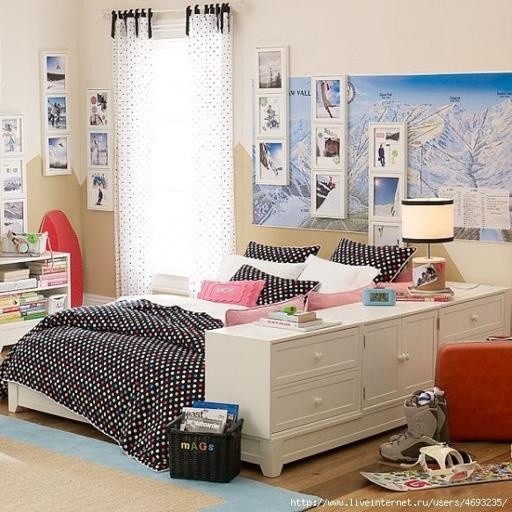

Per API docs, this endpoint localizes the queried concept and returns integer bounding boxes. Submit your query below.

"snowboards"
[359,461,512,492]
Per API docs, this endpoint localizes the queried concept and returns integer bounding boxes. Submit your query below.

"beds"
[1,244,417,447]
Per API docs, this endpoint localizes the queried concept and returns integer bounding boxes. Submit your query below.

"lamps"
[401,196,457,291]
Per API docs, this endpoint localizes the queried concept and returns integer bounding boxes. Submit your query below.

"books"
[259,311,344,332]
[0,257,68,325]
[396,287,455,303]
[180,399,239,435]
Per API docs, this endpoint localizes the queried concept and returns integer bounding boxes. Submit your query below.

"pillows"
[329,239,415,285]
[229,263,319,309]
[225,251,305,287]
[224,295,307,327]
[196,278,264,308]
[297,255,384,297]
[243,239,322,263]
[305,284,377,310]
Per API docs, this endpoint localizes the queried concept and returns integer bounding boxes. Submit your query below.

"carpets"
[0,413,325,512]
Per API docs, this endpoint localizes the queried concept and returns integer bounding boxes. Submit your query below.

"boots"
[379,390,448,463]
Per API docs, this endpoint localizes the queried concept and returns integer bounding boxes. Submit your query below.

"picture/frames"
[255,42,409,247]
[2,49,113,235]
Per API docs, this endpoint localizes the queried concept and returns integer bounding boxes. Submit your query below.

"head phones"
[7,230,29,254]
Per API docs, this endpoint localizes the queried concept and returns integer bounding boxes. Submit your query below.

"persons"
[266,104,273,115]
[378,144,385,167]
[49,102,60,126]
[327,177,334,189]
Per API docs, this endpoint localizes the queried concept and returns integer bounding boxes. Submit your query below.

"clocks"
[362,286,396,306]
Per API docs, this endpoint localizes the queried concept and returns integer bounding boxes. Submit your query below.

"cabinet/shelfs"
[319,301,439,437]
[0,250,73,350]
[395,278,510,346]
[204,323,360,477]
